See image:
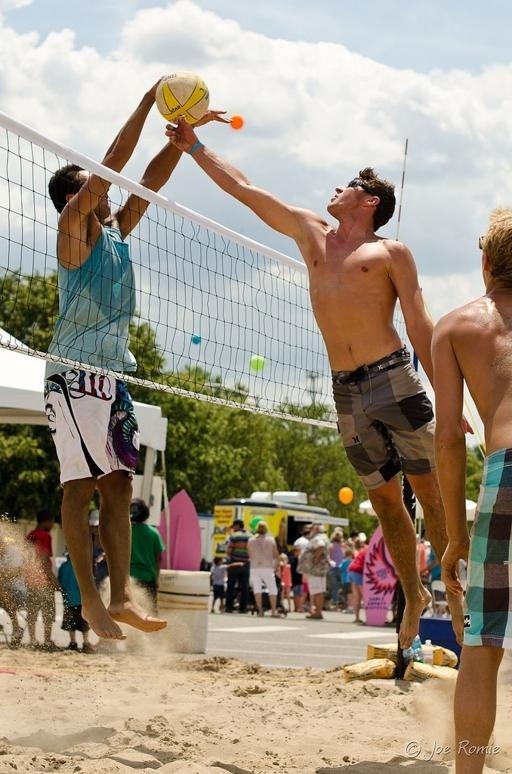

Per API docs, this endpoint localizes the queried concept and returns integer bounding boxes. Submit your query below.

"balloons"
[337,487,354,507]
[190,335,201,346]
[247,354,266,373]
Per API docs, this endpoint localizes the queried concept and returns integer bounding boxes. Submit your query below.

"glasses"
[348,179,379,198]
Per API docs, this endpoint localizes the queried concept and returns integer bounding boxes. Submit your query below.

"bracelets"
[186,139,202,157]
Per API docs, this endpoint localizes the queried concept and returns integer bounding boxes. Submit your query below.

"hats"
[228,519,245,530]
[307,520,328,533]
[87,508,101,529]
[127,495,150,522]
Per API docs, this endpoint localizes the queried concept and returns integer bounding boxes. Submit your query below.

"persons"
[163,114,475,651]
[42,77,232,640]
[430,209,512,774]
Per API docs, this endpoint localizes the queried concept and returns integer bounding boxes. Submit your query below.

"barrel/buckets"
[419,617,462,673]
[156,570,211,653]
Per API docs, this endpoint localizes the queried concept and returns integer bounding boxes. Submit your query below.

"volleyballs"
[156,70,210,123]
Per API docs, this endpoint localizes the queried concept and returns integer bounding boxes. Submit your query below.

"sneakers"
[270,612,281,619]
[67,641,95,654]
[305,610,325,621]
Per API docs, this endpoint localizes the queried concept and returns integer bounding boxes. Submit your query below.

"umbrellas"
[356,496,478,570]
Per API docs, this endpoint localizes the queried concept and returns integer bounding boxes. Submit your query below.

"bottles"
[407,632,432,663]
